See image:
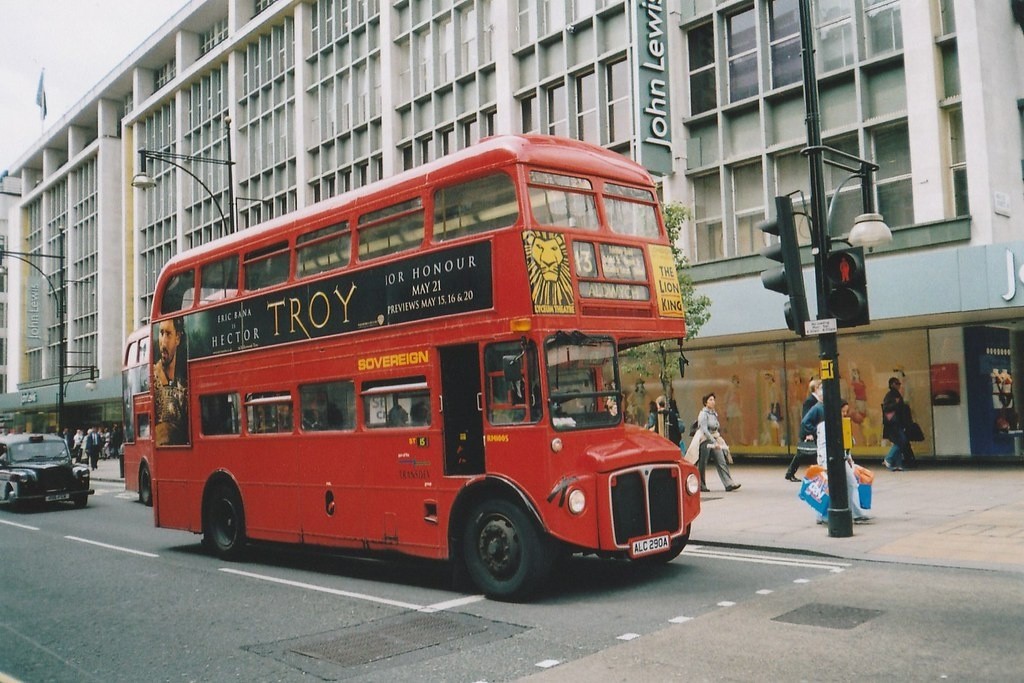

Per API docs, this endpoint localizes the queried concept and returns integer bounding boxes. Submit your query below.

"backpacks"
[690,414,708,436]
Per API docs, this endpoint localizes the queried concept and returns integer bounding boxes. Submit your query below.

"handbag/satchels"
[907,422,924,442]
[851,463,874,510]
[799,464,833,517]
[798,439,818,460]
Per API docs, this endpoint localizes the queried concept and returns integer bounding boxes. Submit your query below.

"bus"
[122,134,701,603]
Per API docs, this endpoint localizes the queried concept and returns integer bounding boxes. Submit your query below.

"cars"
[0,435,95,510]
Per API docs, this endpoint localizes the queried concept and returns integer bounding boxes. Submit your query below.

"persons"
[642,399,657,433]
[1000,369,1014,409]
[789,369,808,446]
[151,316,190,445]
[722,374,749,449]
[764,374,782,446]
[696,391,742,492]
[802,381,826,434]
[815,398,877,526]
[837,373,850,400]
[880,377,913,472]
[847,367,879,447]
[784,379,819,483]
[991,368,1006,410]
[228,361,635,437]
[656,396,682,446]
[892,364,913,409]
[1,423,125,472]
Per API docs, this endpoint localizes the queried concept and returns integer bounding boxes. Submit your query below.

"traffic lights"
[830,248,870,329]
[756,195,811,337]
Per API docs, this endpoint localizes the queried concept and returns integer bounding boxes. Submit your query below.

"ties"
[93,433,96,444]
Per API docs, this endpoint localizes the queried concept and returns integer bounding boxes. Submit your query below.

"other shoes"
[882,459,893,470]
[895,465,903,471]
[726,484,741,492]
[700,486,709,492]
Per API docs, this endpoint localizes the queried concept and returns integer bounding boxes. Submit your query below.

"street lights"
[793,0,891,537]
[130,116,236,235]
[0,225,100,435]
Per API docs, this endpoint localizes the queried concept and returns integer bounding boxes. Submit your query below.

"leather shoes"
[785,474,801,482]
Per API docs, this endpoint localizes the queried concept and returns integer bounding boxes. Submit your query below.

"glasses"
[893,381,901,385]
